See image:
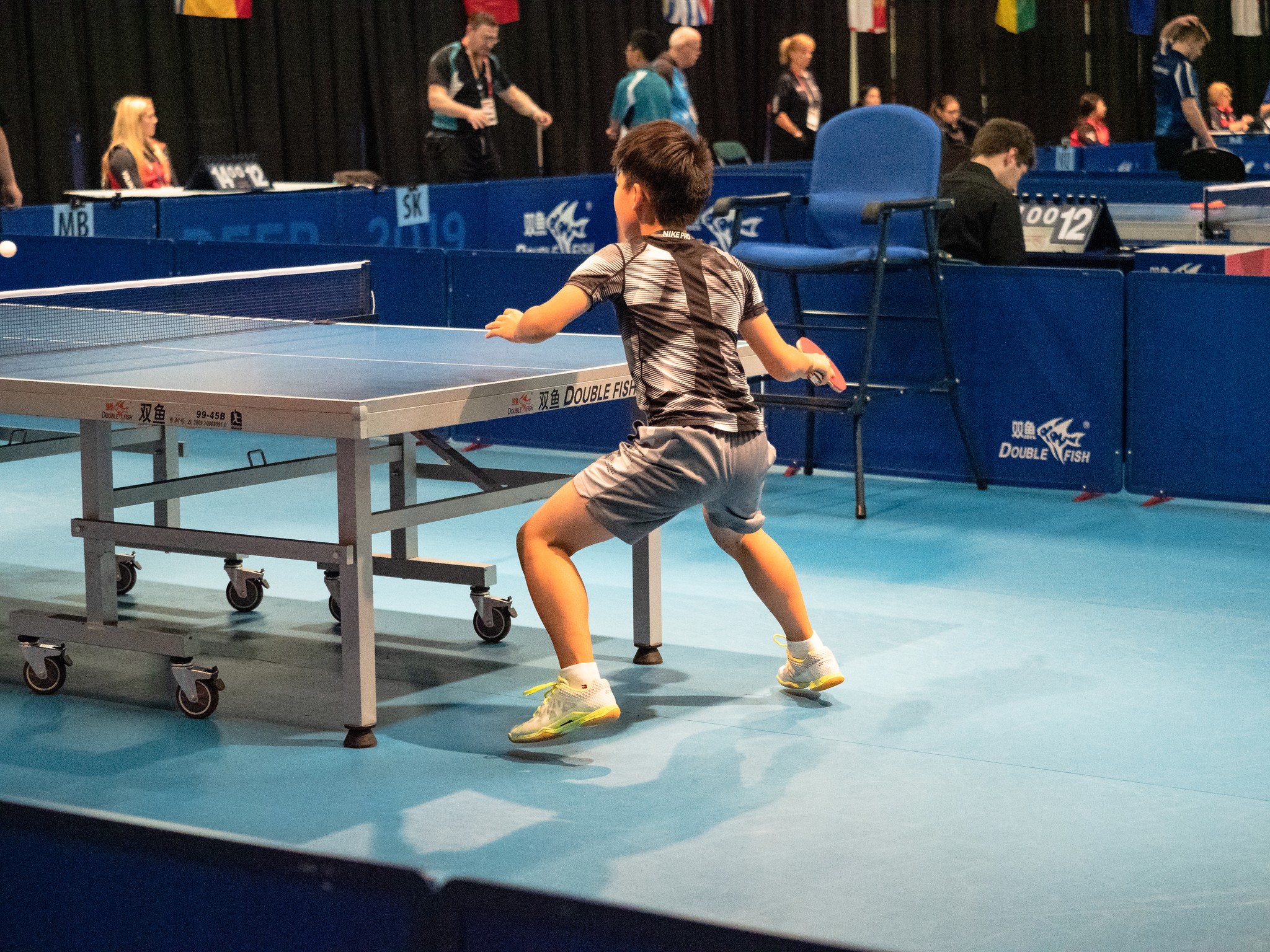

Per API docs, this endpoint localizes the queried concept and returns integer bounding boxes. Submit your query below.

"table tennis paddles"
[796,337,848,393]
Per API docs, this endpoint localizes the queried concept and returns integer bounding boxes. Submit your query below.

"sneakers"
[773,634,844,691]
[507,676,621,743]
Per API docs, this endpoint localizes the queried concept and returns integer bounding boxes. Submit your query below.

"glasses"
[471,30,500,44]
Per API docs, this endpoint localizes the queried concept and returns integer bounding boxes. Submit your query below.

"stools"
[715,104,989,517]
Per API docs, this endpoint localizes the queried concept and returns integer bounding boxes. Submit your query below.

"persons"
[1260,81,1270,117]
[0,126,22,207]
[1208,82,1254,131]
[652,26,702,139]
[1152,15,1217,172]
[1070,93,1110,147]
[856,85,880,108]
[927,92,968,149]
[936,117,1037,265]
[770,33,821,162]
[423,13,552,185]
[101,96,180,190]
[606,29,673,144]
[486,119,846,744]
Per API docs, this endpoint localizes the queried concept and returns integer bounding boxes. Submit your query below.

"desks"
[0,258,769,748]
[1106,180,1270,242]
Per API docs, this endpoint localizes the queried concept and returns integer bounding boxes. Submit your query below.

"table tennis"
[0,240,17,258]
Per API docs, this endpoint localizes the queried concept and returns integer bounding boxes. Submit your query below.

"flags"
[847,0,888,34]
[662,0,713,26]
[994,0,1036,34]
[1125,0,1155,34]
[465,0,520,24]
[1231,0,1262,36]
[175,0,252,18]
